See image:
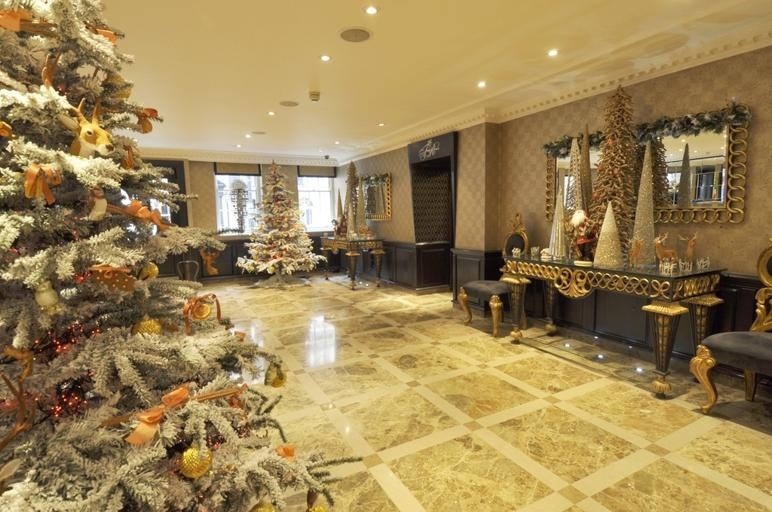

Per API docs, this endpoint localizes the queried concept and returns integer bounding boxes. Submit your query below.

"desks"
[319,235,386,290]
[498,253,729,398]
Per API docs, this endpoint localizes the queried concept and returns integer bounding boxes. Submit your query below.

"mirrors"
[543,105,750,225]
[356,172,393,220]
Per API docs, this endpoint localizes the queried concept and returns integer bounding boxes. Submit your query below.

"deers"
[41,52,115,159]
[678,230,698,272]
[651,231,679,272]
[627,234,644,272]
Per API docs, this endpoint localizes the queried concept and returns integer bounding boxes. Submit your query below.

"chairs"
[177,260,200,281]
[457,213,528,337]
[688,244,772,416]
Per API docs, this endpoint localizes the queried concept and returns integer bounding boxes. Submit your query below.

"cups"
[658,256,710,276]
[530,247,540,256]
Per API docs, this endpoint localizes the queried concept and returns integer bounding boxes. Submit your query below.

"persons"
[569,208,599,266]
